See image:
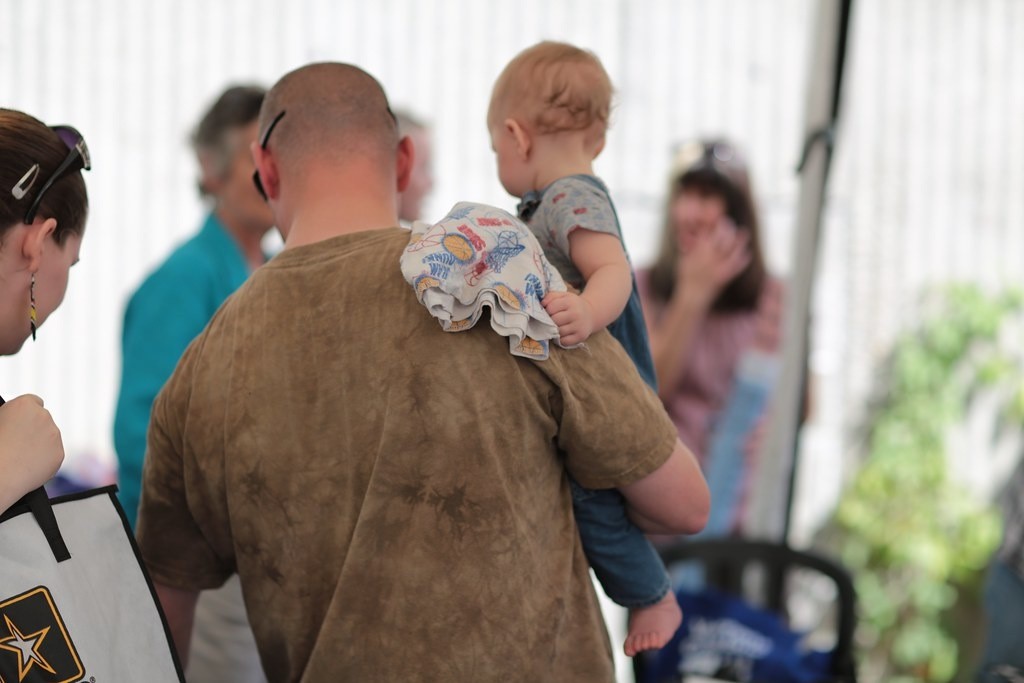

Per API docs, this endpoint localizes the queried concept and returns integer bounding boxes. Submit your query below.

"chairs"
[627,539,857,683]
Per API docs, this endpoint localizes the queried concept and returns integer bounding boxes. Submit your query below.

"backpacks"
[0,396,183,683]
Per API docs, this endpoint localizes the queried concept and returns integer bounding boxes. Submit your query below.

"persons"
[136,62,710,683]
[635,142,812,683]
[395,109,434,222]
[0,107,88,518]
[112,85,267,683]
[487,41,684,657]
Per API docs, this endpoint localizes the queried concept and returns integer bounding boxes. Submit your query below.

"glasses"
[25,125,92,225]
[253,110,286,201]
